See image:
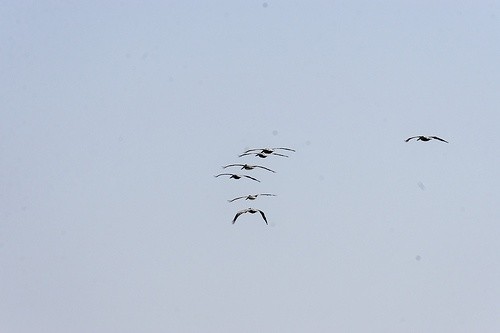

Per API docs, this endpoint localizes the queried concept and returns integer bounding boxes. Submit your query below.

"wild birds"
[215,173,260,183]
[238,147,295,158]
[404,135,448,143]
[232,207,268,226]
[222,163,276,174]
[227,193,276,202]
